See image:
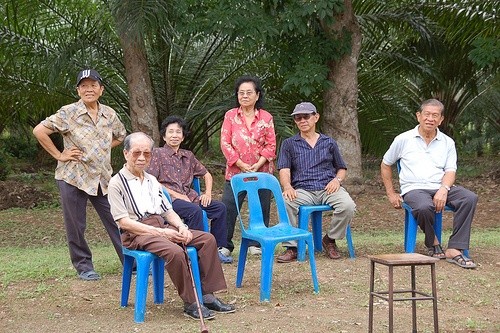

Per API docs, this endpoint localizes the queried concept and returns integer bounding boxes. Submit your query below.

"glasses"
[128,150,153,158]
[294,113,313,121]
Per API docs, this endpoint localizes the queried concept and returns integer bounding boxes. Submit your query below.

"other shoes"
[204,298,235,313]
[217,246,230,256]
[277,247,297,262]
[218,251,234,263]
[181,301,215,320]
[247,245,262,254]
[322,233,341,258]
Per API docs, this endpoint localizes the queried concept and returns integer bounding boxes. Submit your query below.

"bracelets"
[336,176,343,185]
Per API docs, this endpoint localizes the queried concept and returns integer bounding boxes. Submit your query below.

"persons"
[148,116,232,263]
[217,77,276,257]
[381,99,477,269]
[32,70,154,281]
[277,102,355,264]
[107,131,235,321]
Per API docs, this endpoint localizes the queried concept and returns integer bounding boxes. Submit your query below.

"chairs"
[119,185,202,321]
[231,172,318,302]
[396,160,468,258]
[191,177,209,233]
[297,204,354,261]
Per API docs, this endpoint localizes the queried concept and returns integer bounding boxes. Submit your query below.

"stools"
[368,253,440,333]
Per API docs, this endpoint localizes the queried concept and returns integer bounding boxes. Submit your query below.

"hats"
[290,102,317,116]
[76,69,103,86]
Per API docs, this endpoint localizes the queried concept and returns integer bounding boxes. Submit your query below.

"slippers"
[426,244,446,258]
[446,251,477,268]
[77,269,101,279]
[131,266,152,275]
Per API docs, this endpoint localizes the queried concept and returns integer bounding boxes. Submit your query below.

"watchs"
[442,184,450,191]
